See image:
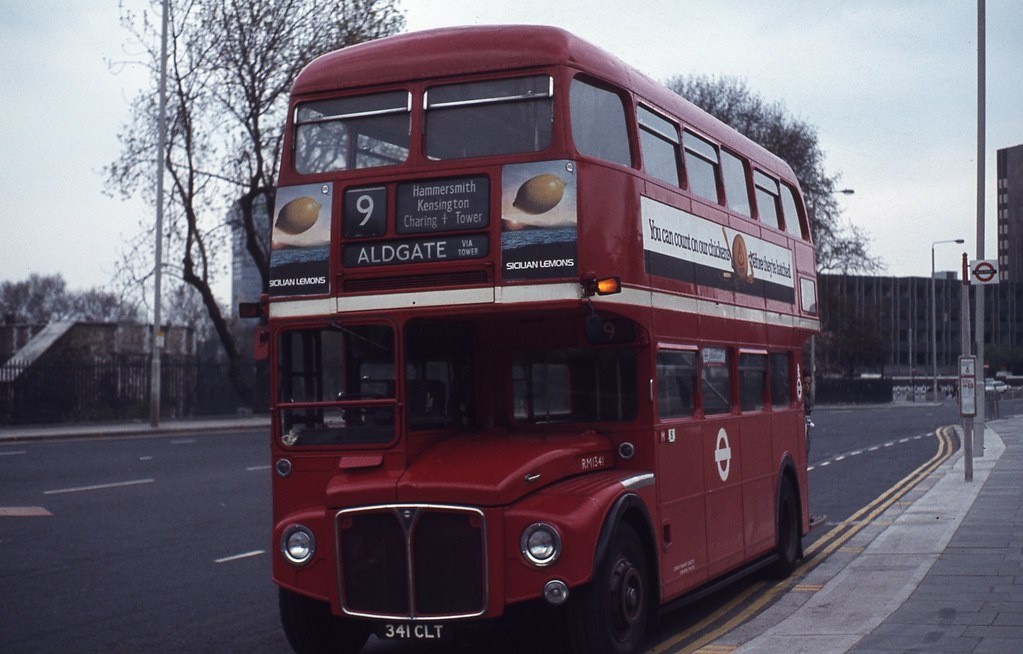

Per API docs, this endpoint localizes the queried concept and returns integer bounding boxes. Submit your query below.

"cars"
[986,381,1011,393]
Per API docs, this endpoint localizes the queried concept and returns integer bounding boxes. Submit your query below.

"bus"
[231,25,820,654]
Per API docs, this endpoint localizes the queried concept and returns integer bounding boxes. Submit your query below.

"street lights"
[811,189,854,244]
[931,239,965,404]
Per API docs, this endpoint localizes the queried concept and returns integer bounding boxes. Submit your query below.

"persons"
[424,355,487,429]
[774,369,812,416]
